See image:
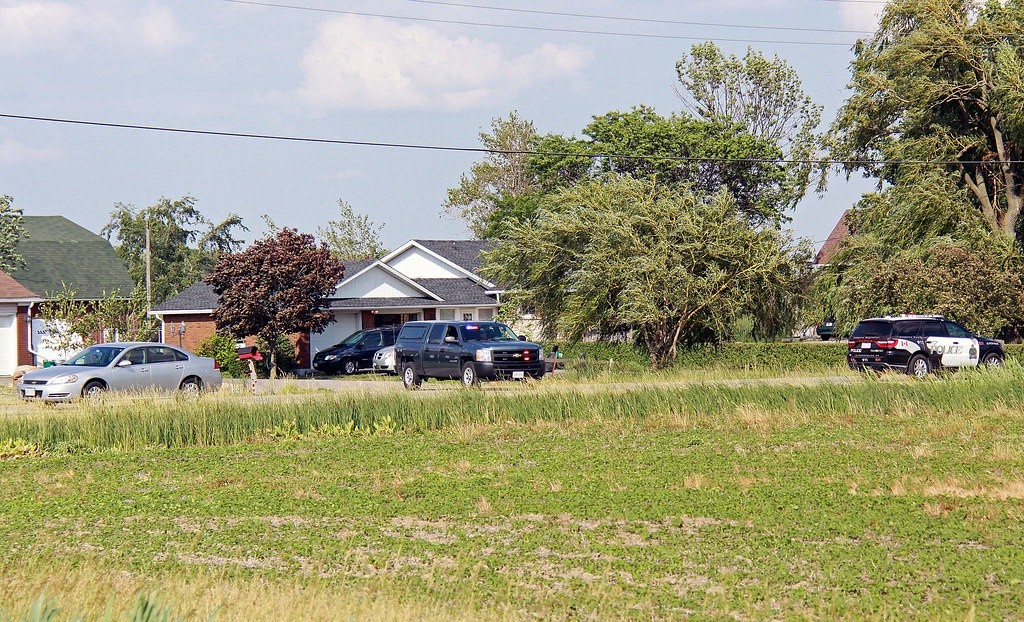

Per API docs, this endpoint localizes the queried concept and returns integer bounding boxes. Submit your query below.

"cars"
[371,343,397,376]
[234,339,247,350]
[816,309,851,341]
[16,342,224,407]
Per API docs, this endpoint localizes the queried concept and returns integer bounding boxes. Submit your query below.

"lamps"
[24,313,31,324]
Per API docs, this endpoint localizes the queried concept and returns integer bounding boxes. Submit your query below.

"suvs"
[847,314,1006,382]
[312,325,403,375]
[393,320,547,391]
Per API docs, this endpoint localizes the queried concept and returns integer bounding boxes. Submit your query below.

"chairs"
[129,349,144,364]
[151,352,174,361]
[113,351,127,362]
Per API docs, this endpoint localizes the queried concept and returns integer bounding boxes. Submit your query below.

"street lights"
[170,320,187,348]
